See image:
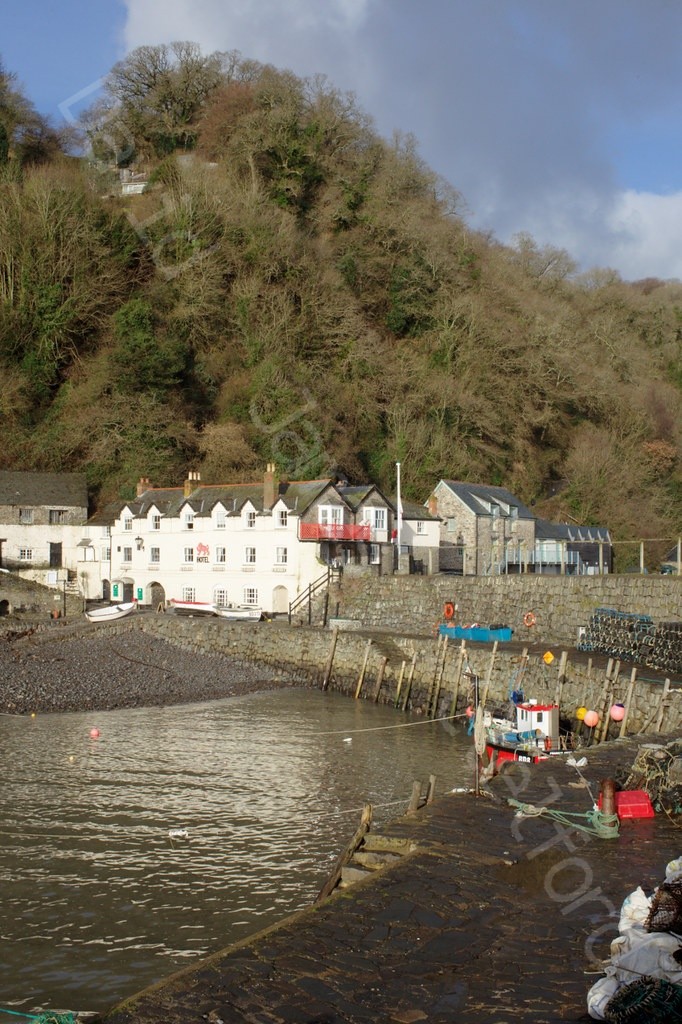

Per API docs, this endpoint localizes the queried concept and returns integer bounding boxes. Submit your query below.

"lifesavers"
[543,738,551,750]
[444,604,454,618]
[523,612,535,627]
[447,622,454,628]
[462,624,470,628]
[471,623,480,628]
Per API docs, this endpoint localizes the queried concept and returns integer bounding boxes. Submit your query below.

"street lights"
[394,461,403,564]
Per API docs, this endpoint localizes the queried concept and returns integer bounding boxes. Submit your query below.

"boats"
[170,598,213,616]
[439,622,512,643]
[469,701,581,770]
[213,604,263,623]
[84,601,138,623]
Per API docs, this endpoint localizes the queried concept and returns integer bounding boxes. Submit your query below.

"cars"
[661,565,678,575]
